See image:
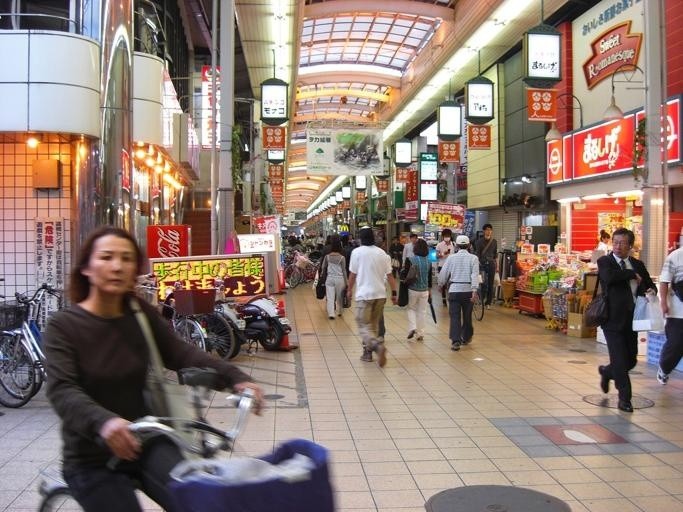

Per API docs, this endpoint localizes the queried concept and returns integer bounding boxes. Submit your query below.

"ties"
[620,260,630,285]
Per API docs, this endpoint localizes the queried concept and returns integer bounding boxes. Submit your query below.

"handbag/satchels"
[130,296,204,460]
[404,257,419,286]
[316,277,326,300]
[391,254,400,270]
[670,276,683,301]
[342,287,351,308]
[584,254,613,328]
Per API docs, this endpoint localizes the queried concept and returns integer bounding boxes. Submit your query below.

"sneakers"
[656,365,670,385]
[461,336,472,344]
[451,340,460,351]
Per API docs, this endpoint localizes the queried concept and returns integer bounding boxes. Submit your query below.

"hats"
[456,235,470,245]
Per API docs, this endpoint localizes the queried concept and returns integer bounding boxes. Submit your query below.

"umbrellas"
[428,294,437,324]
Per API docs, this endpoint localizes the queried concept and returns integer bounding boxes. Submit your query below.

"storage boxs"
[566,311,598,339]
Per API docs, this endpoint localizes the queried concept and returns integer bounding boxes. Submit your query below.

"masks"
[443,238,451,242]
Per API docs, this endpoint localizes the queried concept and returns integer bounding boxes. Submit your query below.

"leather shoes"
[598,365,609,394]
[618,398,634,412]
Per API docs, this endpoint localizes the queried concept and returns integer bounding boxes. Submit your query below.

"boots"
[360,349,373,362]
[372,342,387,368]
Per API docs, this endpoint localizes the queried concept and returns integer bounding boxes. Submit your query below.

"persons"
[349,234,360,248]
[402,233,418,269]
[373,236,386,343]
[388,236,404,279]
[319,240,350,320]
[45,225,265,512]
[474,223,499,310]
[347,225,398,368]
[436,229,456,307]
[400,239,433,341]
[316,233,324,249]
[324,235,334,254]
[591,229,610,263]
[342,235,353,258]
[596,228,658,413]
[656,227,683,385]
[437,234,480,351]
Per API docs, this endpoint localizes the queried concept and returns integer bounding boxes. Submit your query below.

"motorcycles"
[213,274,290,353]
[207,301,246,360]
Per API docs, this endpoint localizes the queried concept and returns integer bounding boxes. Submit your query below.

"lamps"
[545,93,583,141]
[521,174,527,182]
[603,62,644,119]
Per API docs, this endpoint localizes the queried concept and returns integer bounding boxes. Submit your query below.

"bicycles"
[283,250,323,289]
[1,285,61,408]
[138,283,209,361]
[471,285,485,321]
[190,305,235,361]
[35,385,332,512]
